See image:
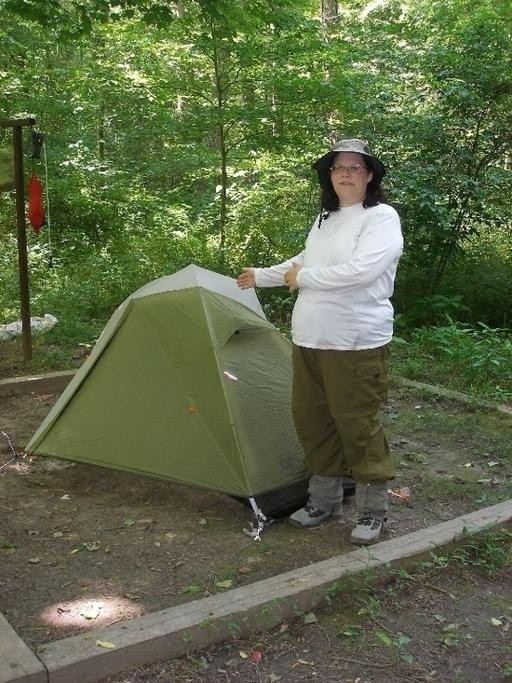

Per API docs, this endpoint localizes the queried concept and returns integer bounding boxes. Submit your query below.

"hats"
[314,139,385,187]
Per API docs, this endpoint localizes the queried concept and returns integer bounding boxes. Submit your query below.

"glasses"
[329,166,365,173]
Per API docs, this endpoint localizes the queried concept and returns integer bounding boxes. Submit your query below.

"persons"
[236,138,406,544]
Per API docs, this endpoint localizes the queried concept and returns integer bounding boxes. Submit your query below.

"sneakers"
[351,518,384,545]
[290,503,343,528]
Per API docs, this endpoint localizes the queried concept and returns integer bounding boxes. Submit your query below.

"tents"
[26,264,357,525]
